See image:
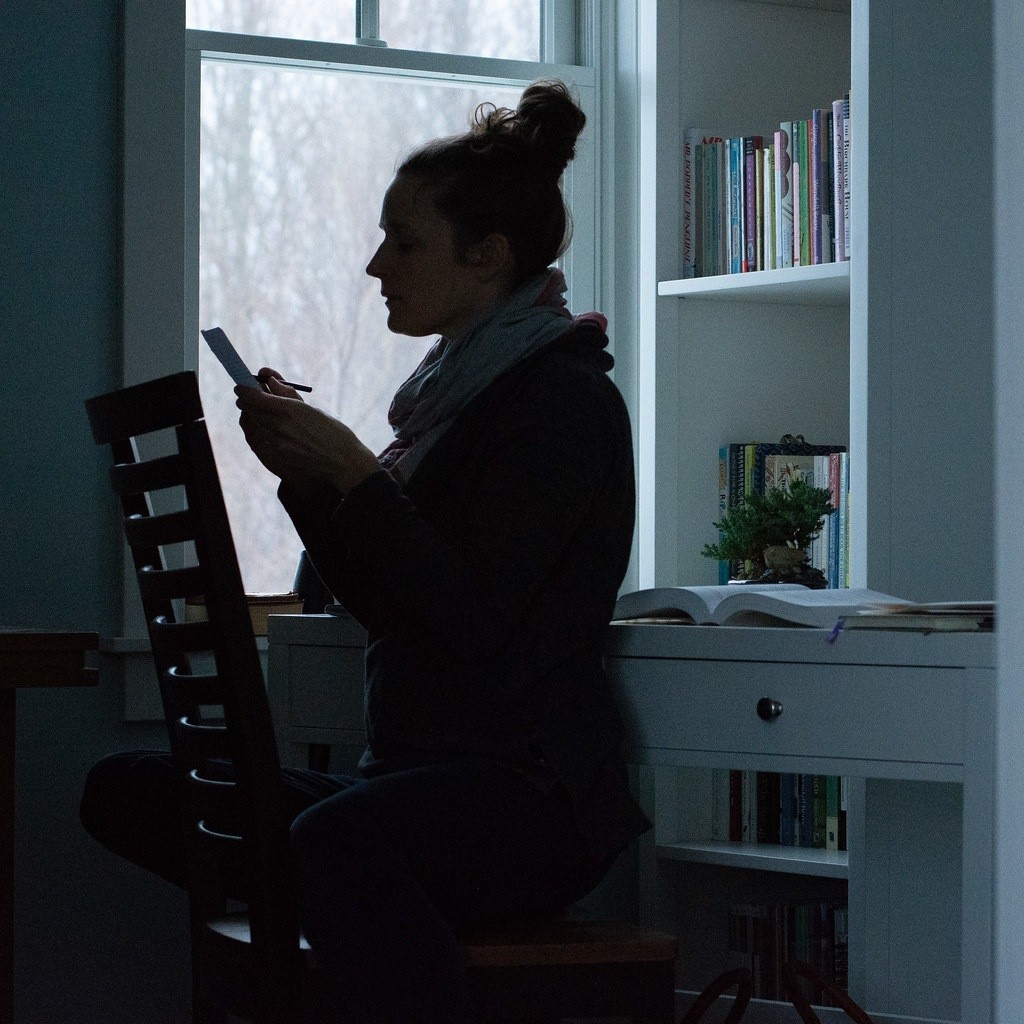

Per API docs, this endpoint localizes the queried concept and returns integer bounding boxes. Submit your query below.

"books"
[667,860,844,997]
[730,770,843,848]
[715,441,853,587]
[681,93,852,272]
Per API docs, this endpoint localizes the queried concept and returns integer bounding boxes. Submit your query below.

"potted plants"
[700,465,838,590]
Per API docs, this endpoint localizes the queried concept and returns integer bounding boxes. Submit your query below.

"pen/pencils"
[252,375,312,393]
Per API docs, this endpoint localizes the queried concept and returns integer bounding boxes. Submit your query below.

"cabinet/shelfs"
[639,0,995,1024]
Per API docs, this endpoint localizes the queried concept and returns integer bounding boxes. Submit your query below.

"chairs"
[86,368,676,1024]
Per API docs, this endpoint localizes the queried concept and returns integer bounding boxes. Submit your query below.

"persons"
[234,85,637,1024]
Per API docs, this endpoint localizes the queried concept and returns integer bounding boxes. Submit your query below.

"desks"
[265,612,998,1024]
